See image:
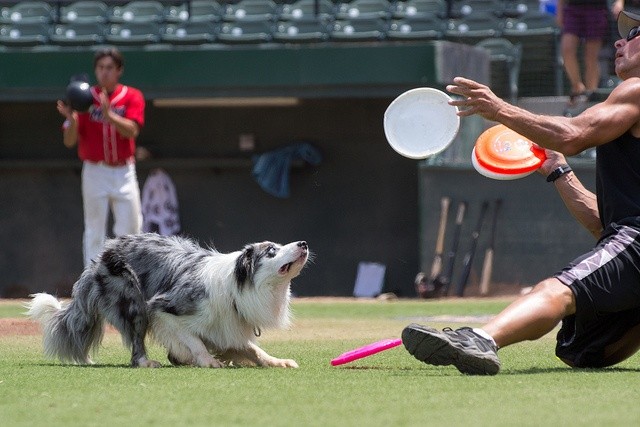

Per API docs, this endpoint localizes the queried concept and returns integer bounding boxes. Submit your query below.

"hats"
[617,11,640,39]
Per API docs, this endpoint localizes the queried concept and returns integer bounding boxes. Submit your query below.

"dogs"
[21,232,317,369]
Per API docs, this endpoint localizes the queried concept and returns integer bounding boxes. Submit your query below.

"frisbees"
[382,87,461,158]
[469,149,537,181]
[332,337,400,365]
[473,123,546,174]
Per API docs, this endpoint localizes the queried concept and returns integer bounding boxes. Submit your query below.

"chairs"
[1,1,564,99]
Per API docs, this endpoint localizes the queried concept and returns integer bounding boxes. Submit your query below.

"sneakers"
[402,324,500,374]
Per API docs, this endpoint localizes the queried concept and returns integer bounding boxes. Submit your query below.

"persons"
[402,27,640,376]
[555,1,623,104]
[56,48,145,269]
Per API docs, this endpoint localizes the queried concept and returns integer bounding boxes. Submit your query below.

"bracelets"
[546,165,572,183]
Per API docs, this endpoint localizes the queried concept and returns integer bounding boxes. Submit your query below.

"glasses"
[627,25,640,41]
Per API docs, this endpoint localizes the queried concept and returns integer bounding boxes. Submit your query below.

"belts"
[92,159,126,167]
